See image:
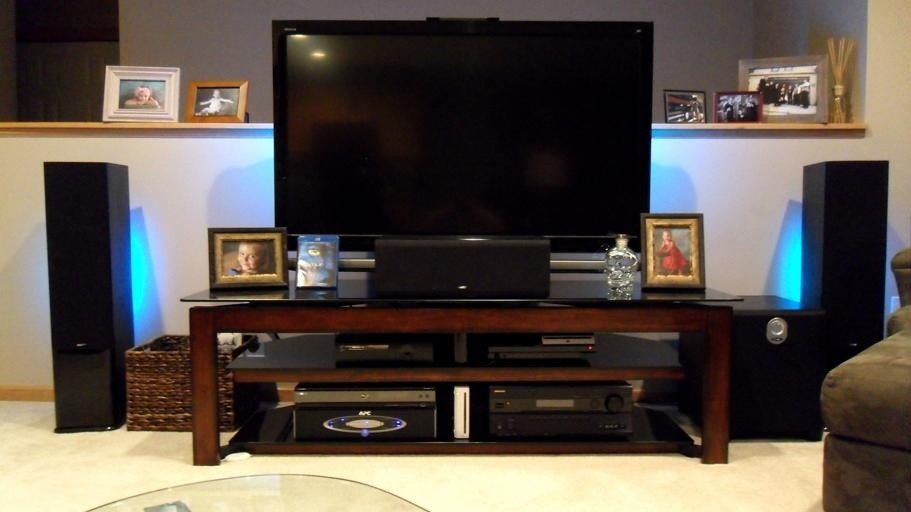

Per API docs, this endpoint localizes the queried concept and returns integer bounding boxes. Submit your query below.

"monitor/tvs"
[271,18,654,272]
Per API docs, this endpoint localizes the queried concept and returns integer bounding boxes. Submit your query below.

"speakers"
[802,159,889,361]
[43,160,134,432]
[373,235,552,298]
[682,295,826,441]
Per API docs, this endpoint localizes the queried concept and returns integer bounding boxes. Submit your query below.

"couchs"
[820,249,911,512]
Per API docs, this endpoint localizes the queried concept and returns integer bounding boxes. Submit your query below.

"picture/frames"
[208,227,289,290]
[102,65,180,123]
[640,213,705,291]
[712,91,763,123]
[664,90,706,123]
[295,234,340,289]
[184,80,248,123]
[738,54,828,124]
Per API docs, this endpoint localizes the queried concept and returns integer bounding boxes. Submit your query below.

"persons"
[195,88,235,117]
[666,76,814,121]
[660,229,687,275]
[605,233,636,302]
[226,239,272,275]
[122,84,159,109]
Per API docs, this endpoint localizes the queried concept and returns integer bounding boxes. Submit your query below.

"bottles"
[607,292,633,300]
[605,234,640,293]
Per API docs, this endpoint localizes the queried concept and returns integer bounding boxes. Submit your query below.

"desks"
[180,280,744,466]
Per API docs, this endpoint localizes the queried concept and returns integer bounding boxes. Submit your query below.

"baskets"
[124,335,257,432]
